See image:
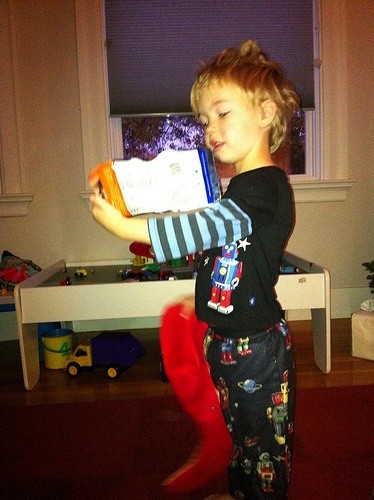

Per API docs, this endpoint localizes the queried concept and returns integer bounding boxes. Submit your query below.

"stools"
[352,309,374,361]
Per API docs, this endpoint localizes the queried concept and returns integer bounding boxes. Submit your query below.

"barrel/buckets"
[41,329,77,370]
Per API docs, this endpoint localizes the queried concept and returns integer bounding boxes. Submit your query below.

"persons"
[86,41,300,500]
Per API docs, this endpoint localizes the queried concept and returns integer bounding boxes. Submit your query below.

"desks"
[14,251,330,391]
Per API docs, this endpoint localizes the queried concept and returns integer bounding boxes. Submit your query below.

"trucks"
[63,331,146,380]
